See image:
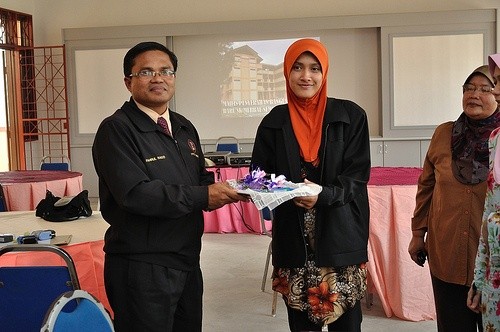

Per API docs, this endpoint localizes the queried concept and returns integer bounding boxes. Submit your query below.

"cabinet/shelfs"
[71,139,431,197]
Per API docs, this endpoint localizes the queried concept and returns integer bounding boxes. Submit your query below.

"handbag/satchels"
[36,189,92,222]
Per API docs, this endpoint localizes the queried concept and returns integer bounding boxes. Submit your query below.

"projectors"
[229,152,253,168]
[203,151,231,165]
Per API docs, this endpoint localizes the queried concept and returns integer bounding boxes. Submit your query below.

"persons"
[92,41,251,332]
[249,38,371,332]
[408,54,500,332]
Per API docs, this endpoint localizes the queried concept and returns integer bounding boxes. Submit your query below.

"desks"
[0,211,115,321]
[0,171,83,212]
[203,151,274,235]
[365,166,439,322]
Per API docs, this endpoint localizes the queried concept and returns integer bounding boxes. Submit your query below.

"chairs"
[216,135,241,154]
[0,155,115,332]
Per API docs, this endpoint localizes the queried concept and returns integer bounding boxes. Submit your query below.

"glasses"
[462,85,495,94]
[127,69,177,80]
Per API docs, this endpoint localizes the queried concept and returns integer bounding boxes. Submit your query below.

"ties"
[157,117,171,135]
